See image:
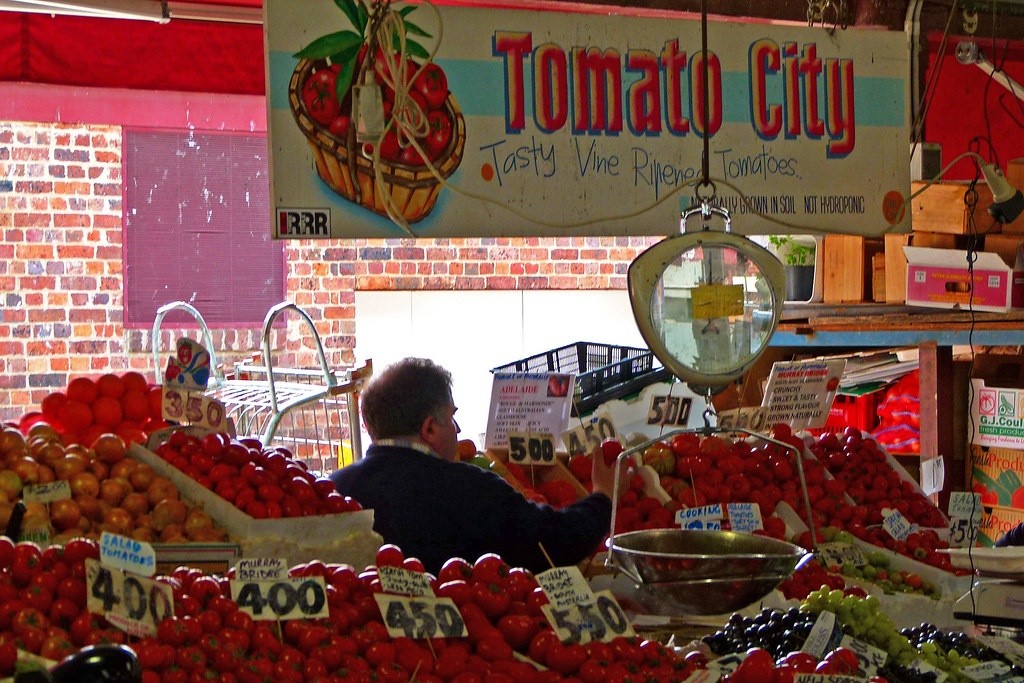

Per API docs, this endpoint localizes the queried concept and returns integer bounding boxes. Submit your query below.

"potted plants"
[767,236,816,302]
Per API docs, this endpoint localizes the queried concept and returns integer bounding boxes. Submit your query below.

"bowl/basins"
[602,527,808,615]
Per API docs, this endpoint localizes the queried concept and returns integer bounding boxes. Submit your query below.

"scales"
[605,181,821,617]
[935,545,1024,627]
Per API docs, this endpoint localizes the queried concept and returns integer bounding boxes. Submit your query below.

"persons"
[329,356,630,580]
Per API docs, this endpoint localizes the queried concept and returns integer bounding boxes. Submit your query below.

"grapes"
[699,585,1024,683]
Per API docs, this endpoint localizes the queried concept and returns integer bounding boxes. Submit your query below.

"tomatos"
[0,372,968,683]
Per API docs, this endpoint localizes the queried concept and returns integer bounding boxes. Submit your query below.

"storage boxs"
[755,179,1024,313]
[87,341,1024,683]
